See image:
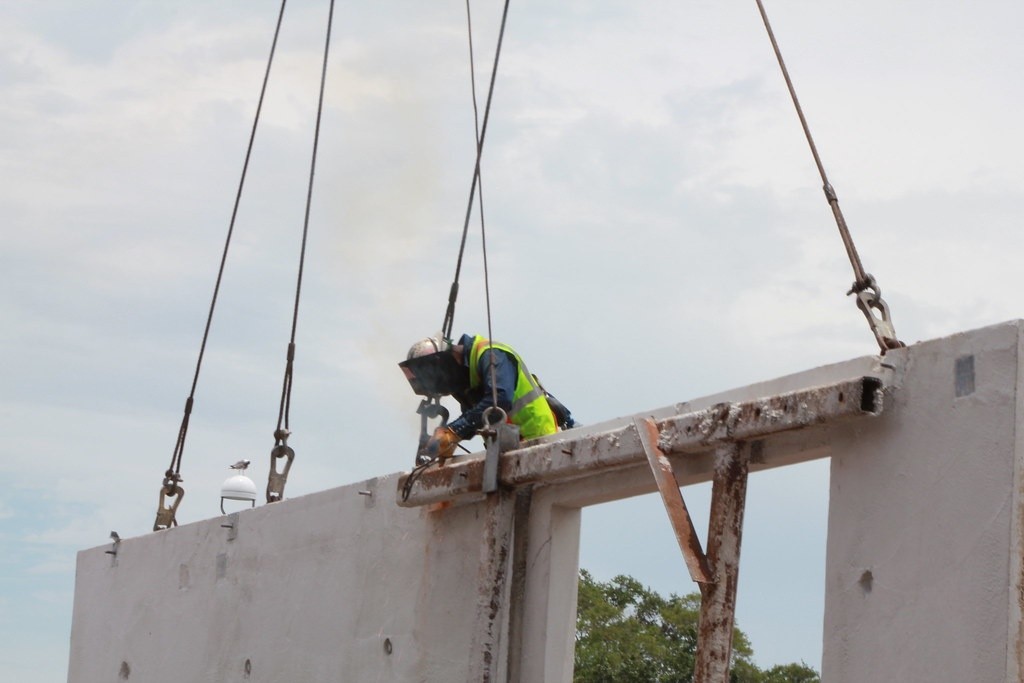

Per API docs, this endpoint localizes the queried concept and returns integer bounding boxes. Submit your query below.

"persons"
[397,332,559,457]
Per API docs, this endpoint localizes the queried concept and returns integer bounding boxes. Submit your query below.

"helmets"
[406,337,449,360]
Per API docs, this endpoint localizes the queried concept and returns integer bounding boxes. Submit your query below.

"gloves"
[425,427,462,467]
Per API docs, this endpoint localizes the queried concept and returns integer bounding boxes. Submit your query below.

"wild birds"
[230,460,250,475]
